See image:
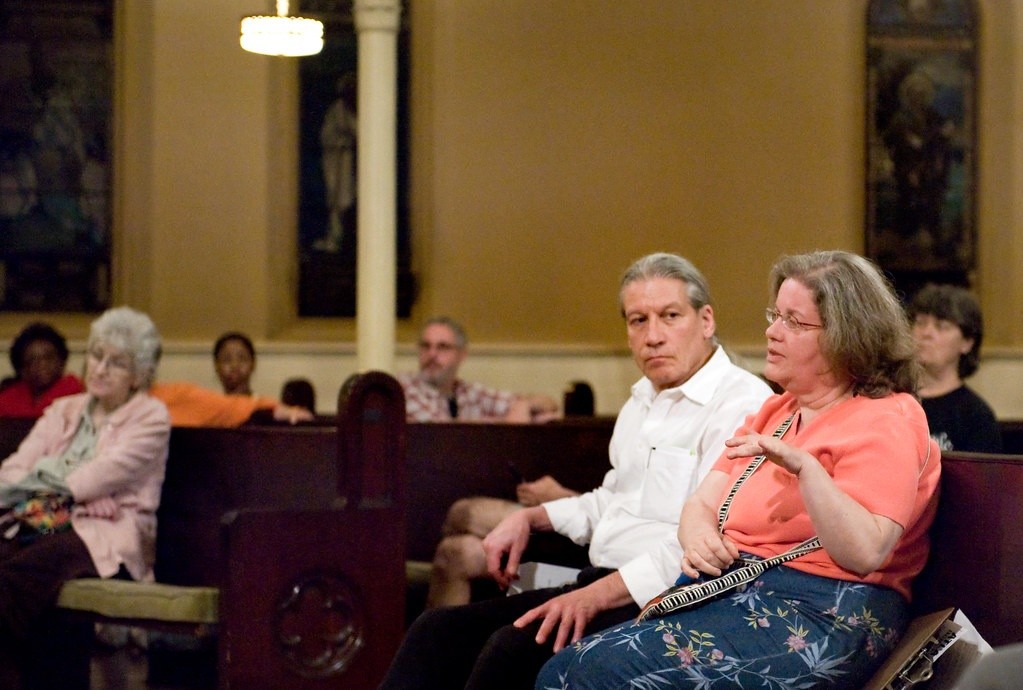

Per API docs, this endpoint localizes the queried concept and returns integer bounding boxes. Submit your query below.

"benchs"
[62,410,1023,690]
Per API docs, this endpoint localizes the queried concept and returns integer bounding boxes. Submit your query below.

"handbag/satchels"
[0,472,73,553]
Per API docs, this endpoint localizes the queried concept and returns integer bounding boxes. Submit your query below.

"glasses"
[764,307,826,333]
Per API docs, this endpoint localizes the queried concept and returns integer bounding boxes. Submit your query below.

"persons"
[382,254,774,690]
[0,320,85,414]
[398,316,558,422]
[913,282,998,454]
[212,334,254,396]
[538,248,945,690]
[0,304,169,689]
[144,344,314,428]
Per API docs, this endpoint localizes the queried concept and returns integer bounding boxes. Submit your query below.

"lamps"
[240,0,325,56]
